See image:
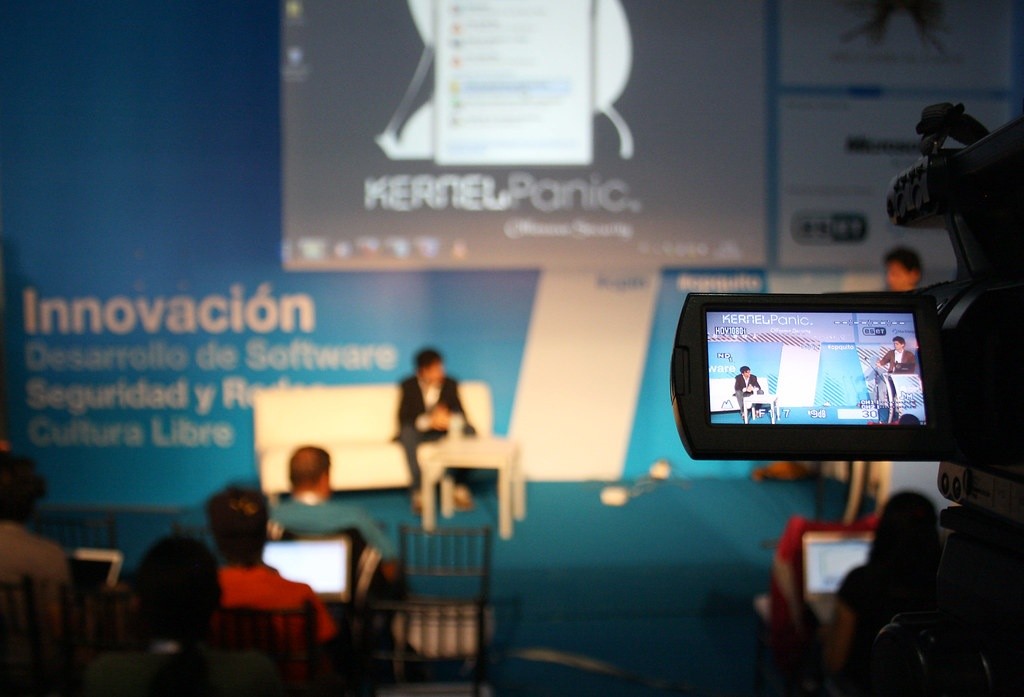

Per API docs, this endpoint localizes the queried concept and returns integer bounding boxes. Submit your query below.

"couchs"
[710,378,770,411]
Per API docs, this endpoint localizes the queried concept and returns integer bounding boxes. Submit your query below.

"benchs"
[254,383,492,506]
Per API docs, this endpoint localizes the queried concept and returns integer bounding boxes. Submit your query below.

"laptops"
[262,535,352,604]
[63,548,123,600]
[802,532,874,622]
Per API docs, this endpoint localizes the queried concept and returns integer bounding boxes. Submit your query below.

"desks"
[743,394,781,425]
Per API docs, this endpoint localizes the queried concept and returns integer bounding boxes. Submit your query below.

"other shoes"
[742,414,745,420]
[411,491,423,515]
[452,486,473,511]
[755,410,759,418]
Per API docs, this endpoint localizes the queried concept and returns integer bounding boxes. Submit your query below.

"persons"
[394,351,474,517]
[0,445,399,697]
[824,492,943,697]
[875,336,916,424]
[735,366,764,419]
[881,245,925,291]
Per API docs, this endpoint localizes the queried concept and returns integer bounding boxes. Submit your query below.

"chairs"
[222,524,495,697]
